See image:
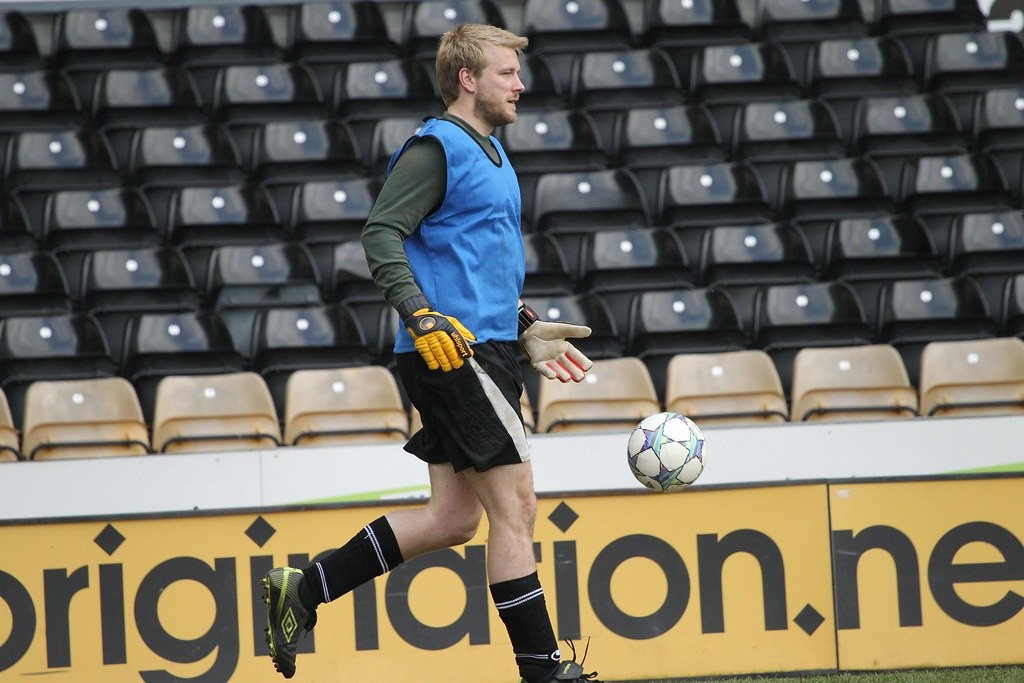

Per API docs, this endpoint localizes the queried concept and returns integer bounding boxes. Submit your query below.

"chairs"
[0,0,1022,462]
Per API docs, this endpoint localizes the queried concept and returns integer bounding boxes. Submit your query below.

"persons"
[262,25,601,683]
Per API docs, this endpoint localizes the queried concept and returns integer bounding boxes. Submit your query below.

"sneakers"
[521,636,604,683]
[261,567,317,679]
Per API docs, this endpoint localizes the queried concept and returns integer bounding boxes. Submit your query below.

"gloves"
[398,295,477,372]
[517,303,593,383]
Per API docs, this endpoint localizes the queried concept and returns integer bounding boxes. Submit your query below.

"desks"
[0,411,1024,683]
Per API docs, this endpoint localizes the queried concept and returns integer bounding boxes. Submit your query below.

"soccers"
[624,410,709,493]
